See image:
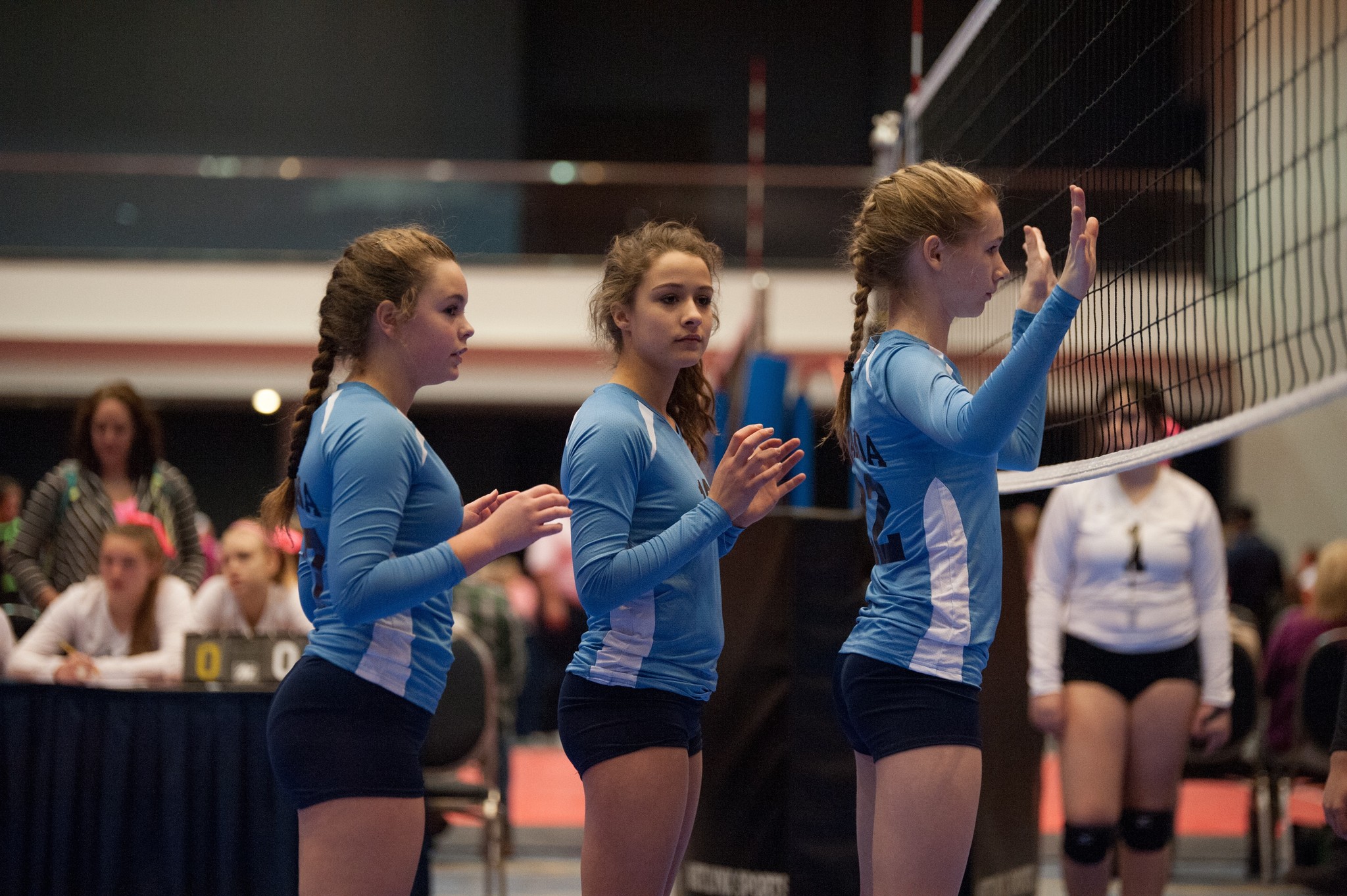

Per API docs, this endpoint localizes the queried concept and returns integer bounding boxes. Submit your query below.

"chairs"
[421,634,506,896]
[1180,618,1347,882]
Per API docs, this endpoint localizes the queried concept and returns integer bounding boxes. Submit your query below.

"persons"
[559,221,806,896]
[0,379,587,739]
[837,159,1100,896]
[265,226,571,894]
[1217,495,1347,896]
[1024,375,1235,896]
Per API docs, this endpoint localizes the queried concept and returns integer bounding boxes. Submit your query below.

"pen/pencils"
[57,639,106,677]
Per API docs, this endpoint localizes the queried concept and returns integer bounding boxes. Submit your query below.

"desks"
[0,674,428,894]
